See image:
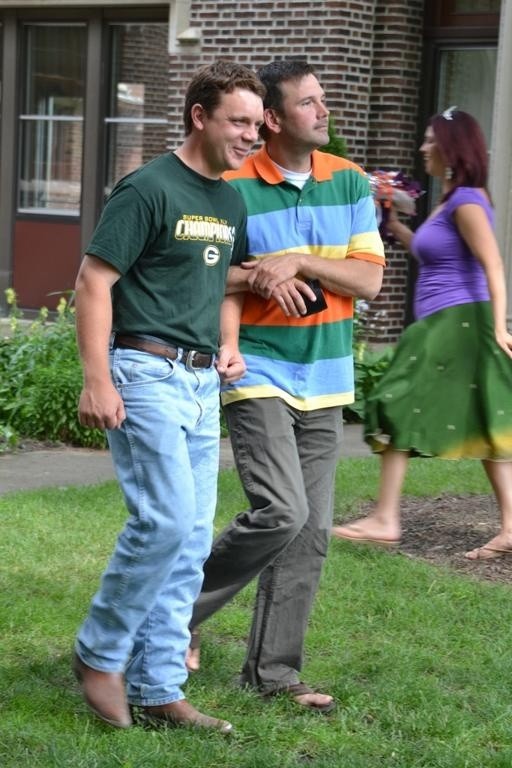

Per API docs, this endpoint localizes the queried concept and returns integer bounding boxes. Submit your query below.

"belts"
[114,333,217,373]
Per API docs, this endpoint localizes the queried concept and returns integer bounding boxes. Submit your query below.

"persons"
[70,58,252,745]
[179,55,389,712]
[329,103,512,565]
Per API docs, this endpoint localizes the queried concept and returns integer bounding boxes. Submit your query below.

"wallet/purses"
[293,279,328,318]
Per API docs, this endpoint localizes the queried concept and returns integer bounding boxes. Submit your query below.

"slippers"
[184,627,203,675]
[464,542,512,562]
[331,522,403,547]
[273,680,337,713]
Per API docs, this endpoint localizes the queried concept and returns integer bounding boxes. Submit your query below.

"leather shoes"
[71,651,135,729]
[129,698,234,734]
